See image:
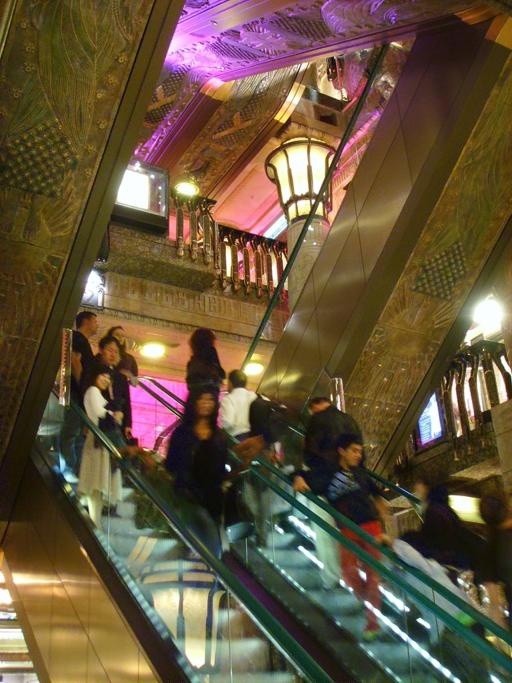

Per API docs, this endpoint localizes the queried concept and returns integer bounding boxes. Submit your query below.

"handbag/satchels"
[110,430,139,461]
[94,411,127,451]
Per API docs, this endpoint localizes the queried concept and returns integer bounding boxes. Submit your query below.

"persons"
[166,383,231,564]
[288,431,396,641]
[479,488,512,636]
[302,395,366,592]
[48,309,140,536]
[217,368,263,500]
[399,470,473,636]
[186,327,226,410]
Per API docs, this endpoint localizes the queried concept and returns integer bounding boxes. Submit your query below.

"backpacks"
[248,391,289,446]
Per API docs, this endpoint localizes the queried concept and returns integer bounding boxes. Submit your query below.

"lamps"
[261,130,337,223]
[168,167,204,206]
